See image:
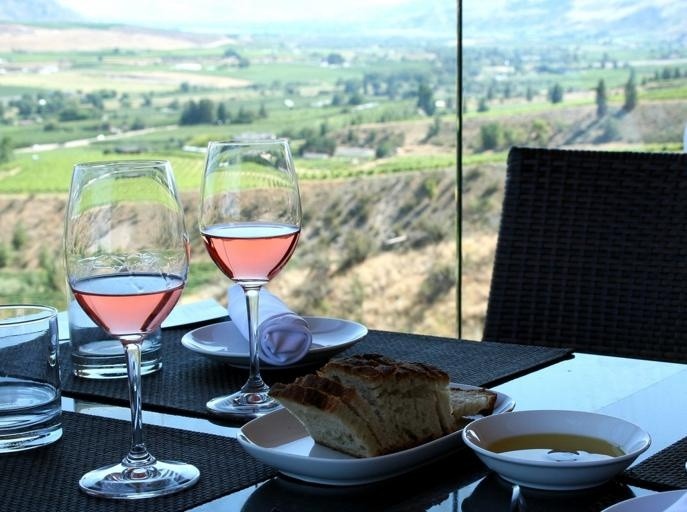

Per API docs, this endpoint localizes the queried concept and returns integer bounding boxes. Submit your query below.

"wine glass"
[66,160,202,500]
[201,140,303,417]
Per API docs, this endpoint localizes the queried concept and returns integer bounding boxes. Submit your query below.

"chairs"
[480,146,687,364]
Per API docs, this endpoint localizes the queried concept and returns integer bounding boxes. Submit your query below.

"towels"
[224,285,311,366]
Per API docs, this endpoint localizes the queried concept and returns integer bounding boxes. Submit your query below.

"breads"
[267,353,497,458]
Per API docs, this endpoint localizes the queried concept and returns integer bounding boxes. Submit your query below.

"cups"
[0,303,62,455]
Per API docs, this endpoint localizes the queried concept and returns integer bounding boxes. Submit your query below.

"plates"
[236,370,517,489]
[460,410,657,497]
[600,490,687,512]
[181,315,368,370]
